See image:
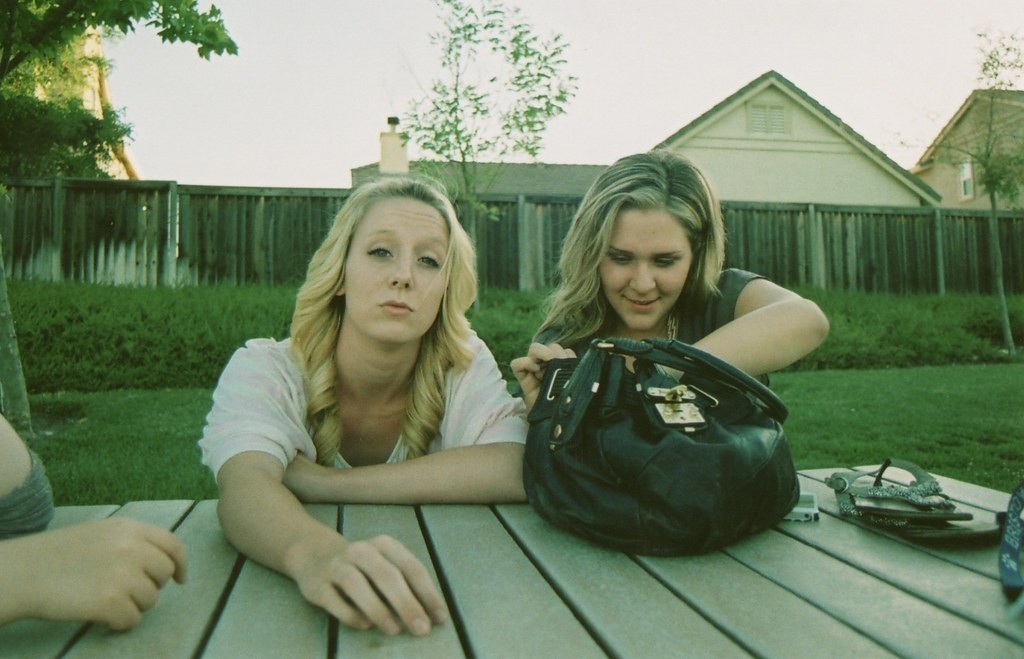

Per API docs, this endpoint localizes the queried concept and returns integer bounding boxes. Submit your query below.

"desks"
[1,462,1024,659]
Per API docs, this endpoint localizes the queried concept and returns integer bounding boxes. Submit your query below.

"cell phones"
[783,493,819,522]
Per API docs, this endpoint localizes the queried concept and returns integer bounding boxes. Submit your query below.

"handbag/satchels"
[522,335,800,559]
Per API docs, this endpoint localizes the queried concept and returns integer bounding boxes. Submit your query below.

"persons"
[0,413,186,634]
[506,147,830,417]
[197,171,531,637]
